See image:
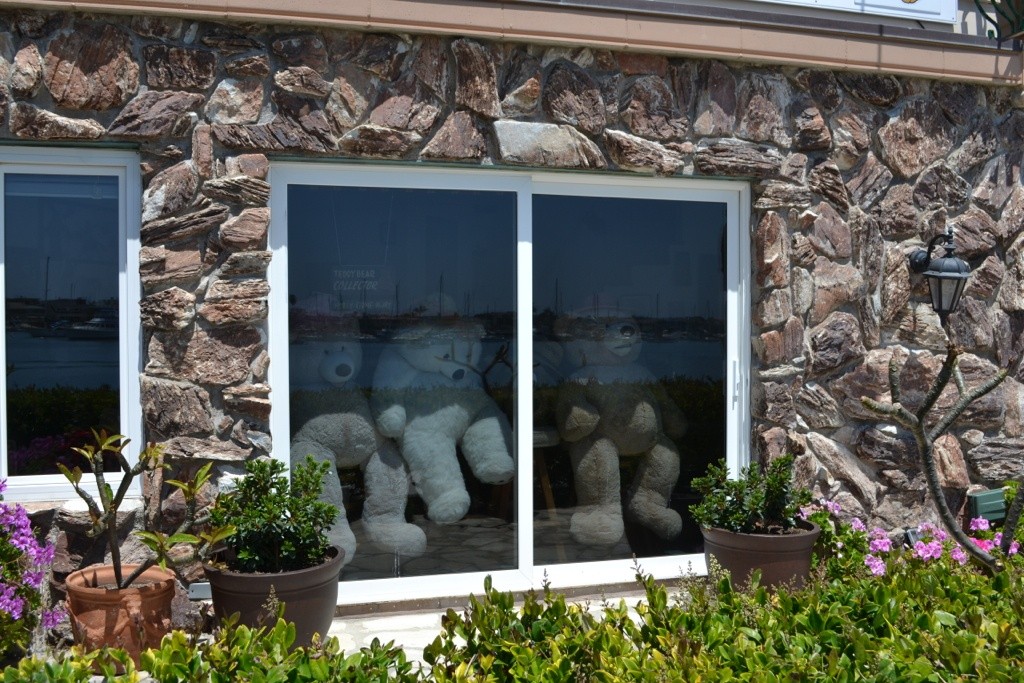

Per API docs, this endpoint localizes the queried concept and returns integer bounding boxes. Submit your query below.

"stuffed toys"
[373,282,517,529]
[281,289,430,567]
[548,278,684,547]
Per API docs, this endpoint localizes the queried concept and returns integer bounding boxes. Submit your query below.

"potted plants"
[55,427,239,681]
[685,447,823,590]
[203,455,347,648]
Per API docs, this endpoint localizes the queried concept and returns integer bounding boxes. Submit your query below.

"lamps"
[908,222,972,315]
